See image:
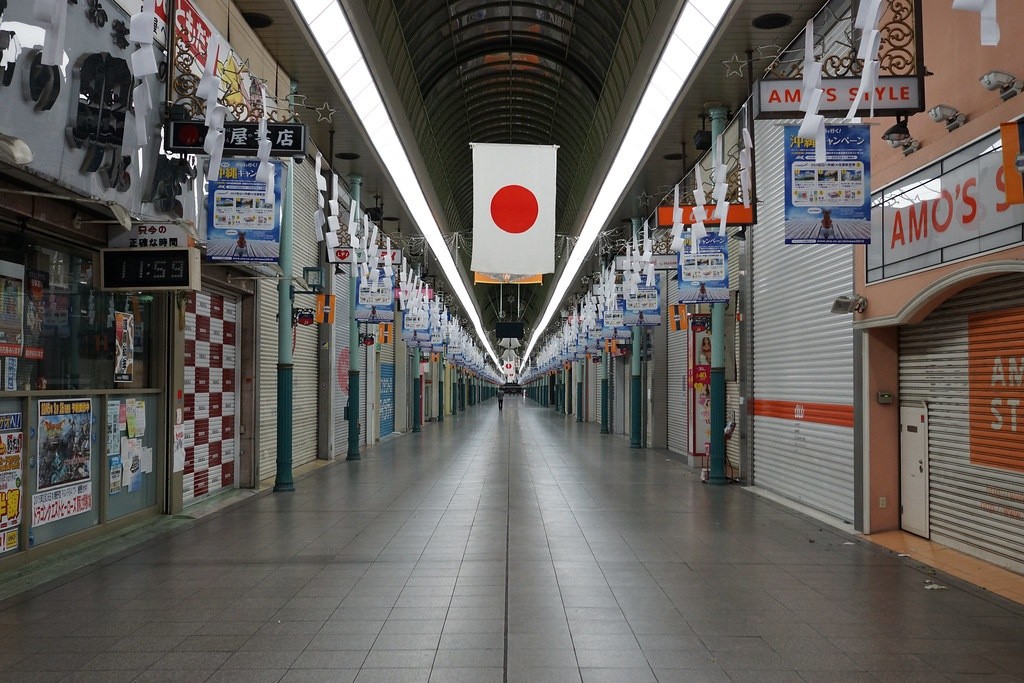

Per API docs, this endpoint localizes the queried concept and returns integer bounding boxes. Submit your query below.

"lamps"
[978,68,1024,100]
[830,294,866,315]
[878,117,911,141]
[615,338,633,349]
[928,103,964,132]
[692,114,712,150]
[365,202,384,220]
[887,137,920,156]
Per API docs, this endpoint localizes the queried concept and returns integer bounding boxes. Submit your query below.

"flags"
[473,271,543,284]
[470,142,557,275]
[504,360,517,380]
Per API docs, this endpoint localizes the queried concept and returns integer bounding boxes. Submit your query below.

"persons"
[497,387,504,411]
